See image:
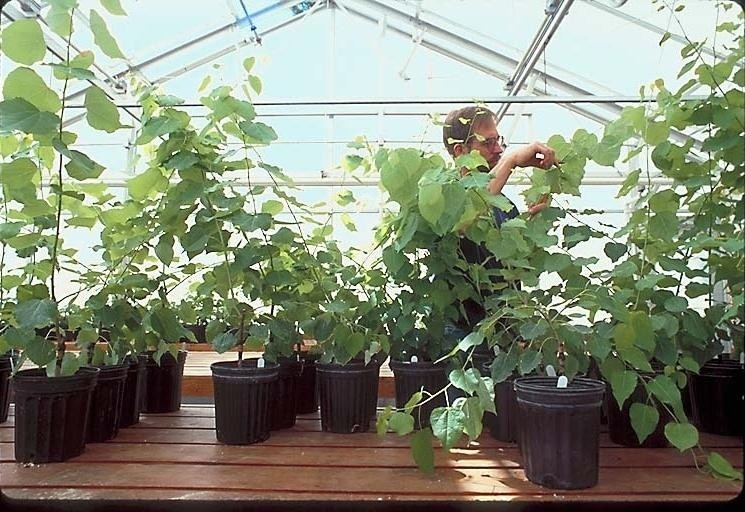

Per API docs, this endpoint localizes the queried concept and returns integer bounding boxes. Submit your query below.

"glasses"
[469,136,500,152]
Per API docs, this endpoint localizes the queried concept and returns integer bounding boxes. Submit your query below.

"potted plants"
[2,2,200,463]
[481,129,607,491]
[187,107,316,442]
[605,103,743,448]
[386,158,458,428]
[315,261,379,438]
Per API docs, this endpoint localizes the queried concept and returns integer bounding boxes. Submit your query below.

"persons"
[426,107,560,405]
